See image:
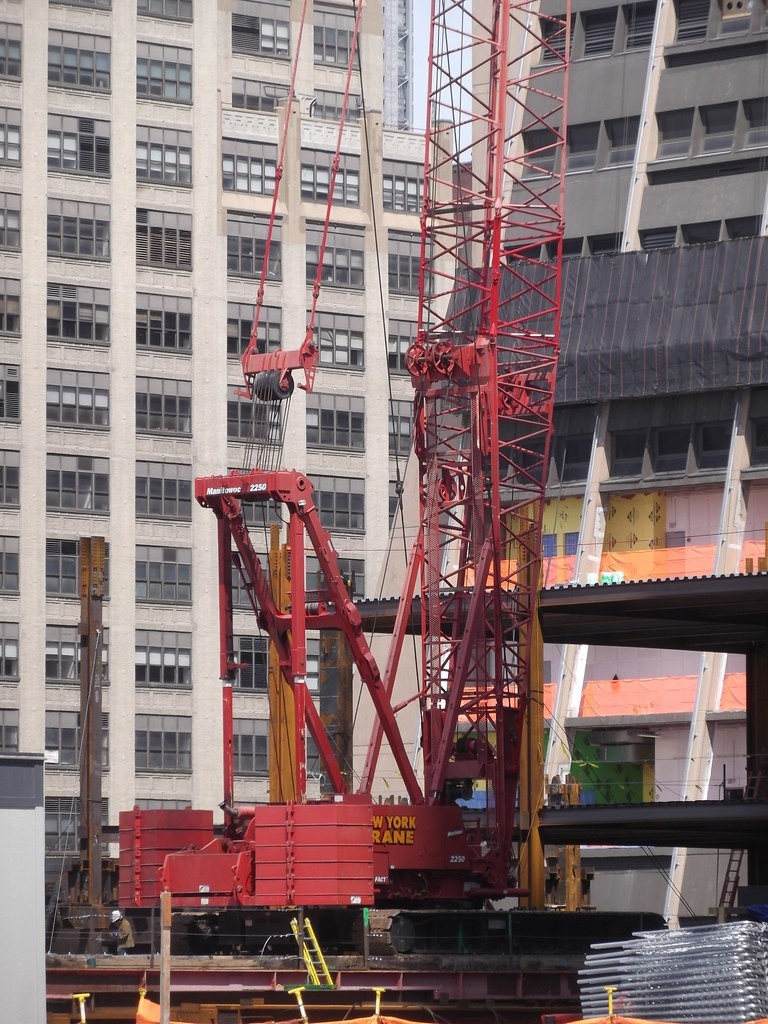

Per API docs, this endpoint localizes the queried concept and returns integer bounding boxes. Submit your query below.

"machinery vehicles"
[117,0,598,977]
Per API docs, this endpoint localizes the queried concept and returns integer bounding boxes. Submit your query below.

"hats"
[112,910,122,921]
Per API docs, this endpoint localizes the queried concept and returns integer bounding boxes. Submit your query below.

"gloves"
[112,932,117,935]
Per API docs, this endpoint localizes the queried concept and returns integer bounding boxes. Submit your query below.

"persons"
[112,911,135,954]
